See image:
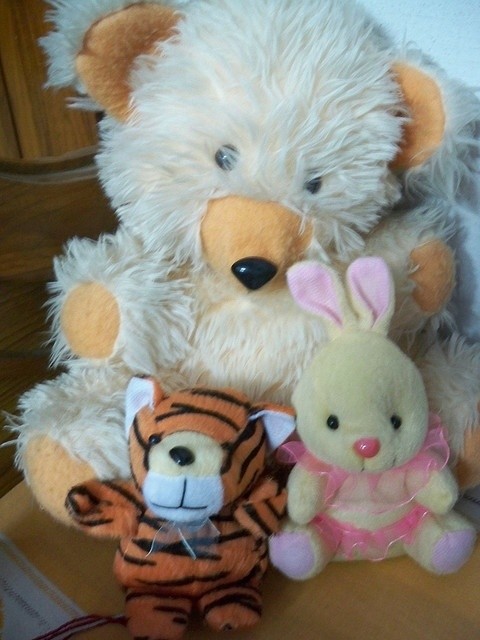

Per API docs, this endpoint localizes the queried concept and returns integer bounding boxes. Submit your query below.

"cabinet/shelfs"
[1,0,99,176]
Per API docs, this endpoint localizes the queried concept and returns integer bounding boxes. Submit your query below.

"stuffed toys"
[268,256,476,580]
[0,0,480,535]
[65,374,297,639]
